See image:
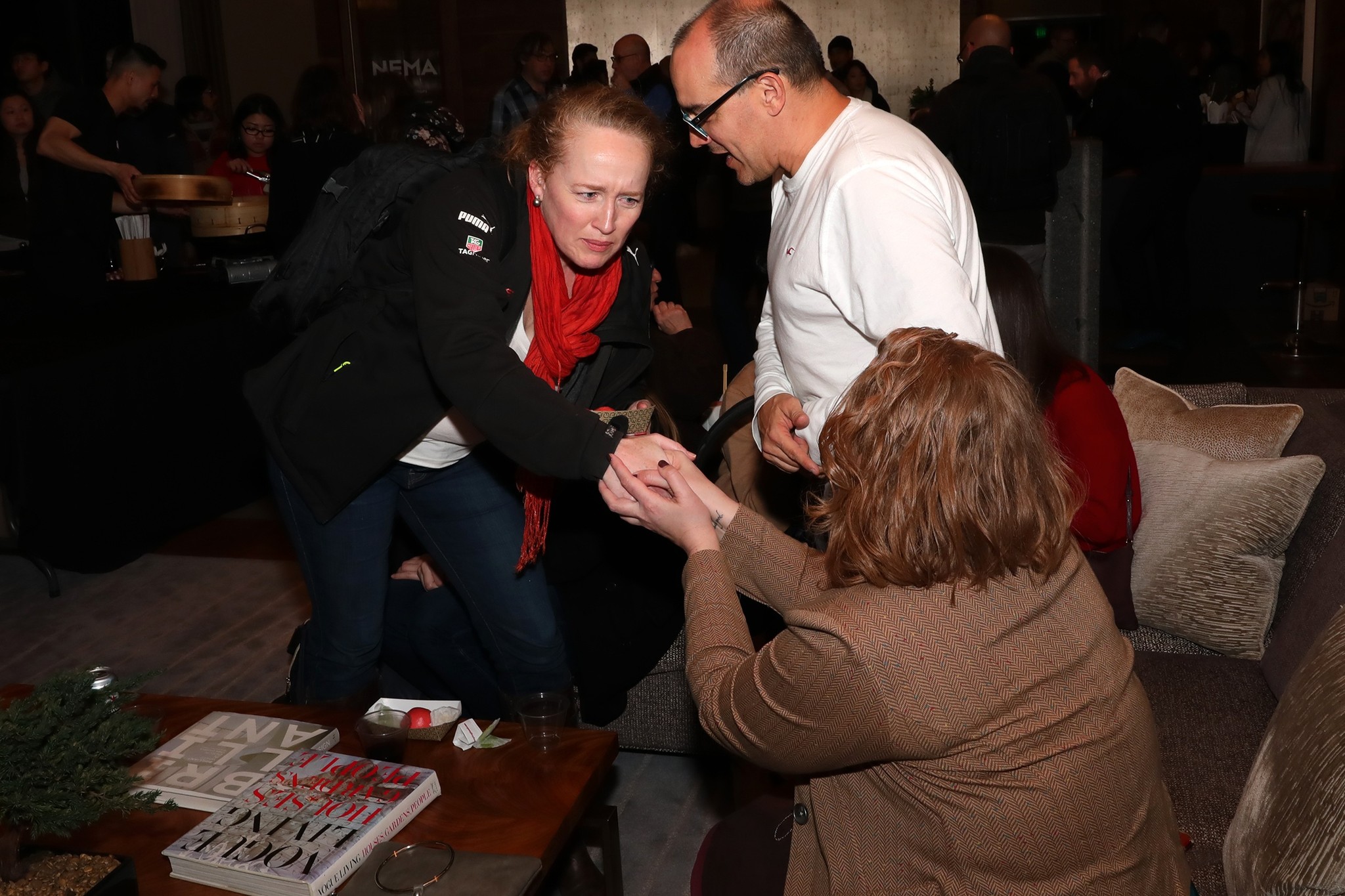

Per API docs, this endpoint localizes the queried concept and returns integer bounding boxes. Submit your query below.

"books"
[162,747,441,896]
[127,710,339,813]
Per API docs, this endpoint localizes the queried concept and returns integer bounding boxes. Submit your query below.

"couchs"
[1121,381,1344,896]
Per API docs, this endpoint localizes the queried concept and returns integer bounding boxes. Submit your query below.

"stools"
[1252,190,1345,368]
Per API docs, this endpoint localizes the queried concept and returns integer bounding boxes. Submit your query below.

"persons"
[908,14,1069,272]
[486,30,563,145]
[599,327,1194,896]
[0,39,464,345]
[668,0,1005,545]
[828,36,879,93]
[1026,10,1313,173]
[711,157,772,374]
[649,268,732,452]
[565,43,608,86]
[843,62,890,112]
[242,85,698,719]
[983,247,1142,552]
[380,477,686,722]
[716,358,793,532]
[815,47,851,96]
[610,34,703,305]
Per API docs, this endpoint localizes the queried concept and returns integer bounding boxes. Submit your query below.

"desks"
[1,680,622,896]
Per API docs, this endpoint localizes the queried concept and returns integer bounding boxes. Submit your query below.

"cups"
[352,709,411,764]
[517,692,570,750]
[118,237,157,280]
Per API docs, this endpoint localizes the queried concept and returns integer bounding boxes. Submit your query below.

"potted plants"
[1,664,166,896]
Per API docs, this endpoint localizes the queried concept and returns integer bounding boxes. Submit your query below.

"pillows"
[1223,605,1345,896]
[1127,438,1325,661]
[1113,368,1305,460]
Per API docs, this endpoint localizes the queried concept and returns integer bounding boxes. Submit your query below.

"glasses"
[241,123,275,137]
[531,53,558,63]
[681,67,781,138]
[610,53,638,65]
